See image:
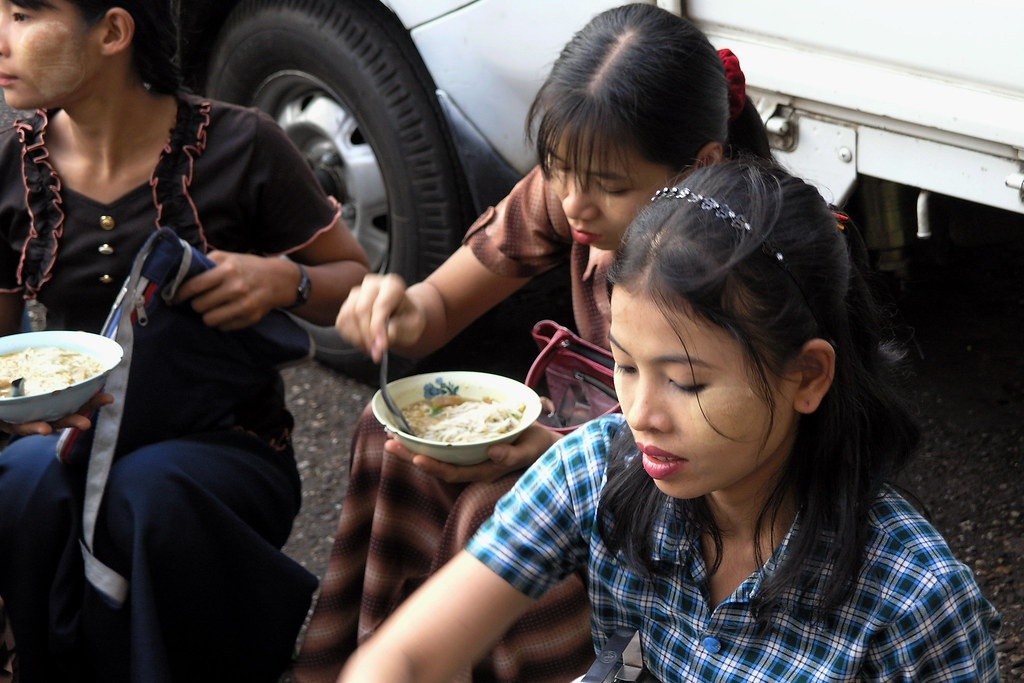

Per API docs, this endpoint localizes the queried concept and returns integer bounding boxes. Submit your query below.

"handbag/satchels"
[524,318,622,435]
[55,226,312,487]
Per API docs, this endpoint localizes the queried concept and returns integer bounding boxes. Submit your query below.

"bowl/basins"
[0,332,124,423]
[373,372,542,466]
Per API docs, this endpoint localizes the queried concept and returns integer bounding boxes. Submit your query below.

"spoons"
[379,315,416,436]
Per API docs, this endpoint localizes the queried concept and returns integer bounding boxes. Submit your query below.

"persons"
[282,4,771,683]
[0,0,373,683]
[334,155,1003,683]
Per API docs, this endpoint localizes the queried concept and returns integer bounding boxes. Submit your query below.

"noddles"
[402,394,526,442]
[0,346,100,395]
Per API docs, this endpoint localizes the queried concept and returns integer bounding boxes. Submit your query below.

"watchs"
[295,264,311,306]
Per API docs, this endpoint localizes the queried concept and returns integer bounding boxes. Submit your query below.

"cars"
[126,0,1024,380]
